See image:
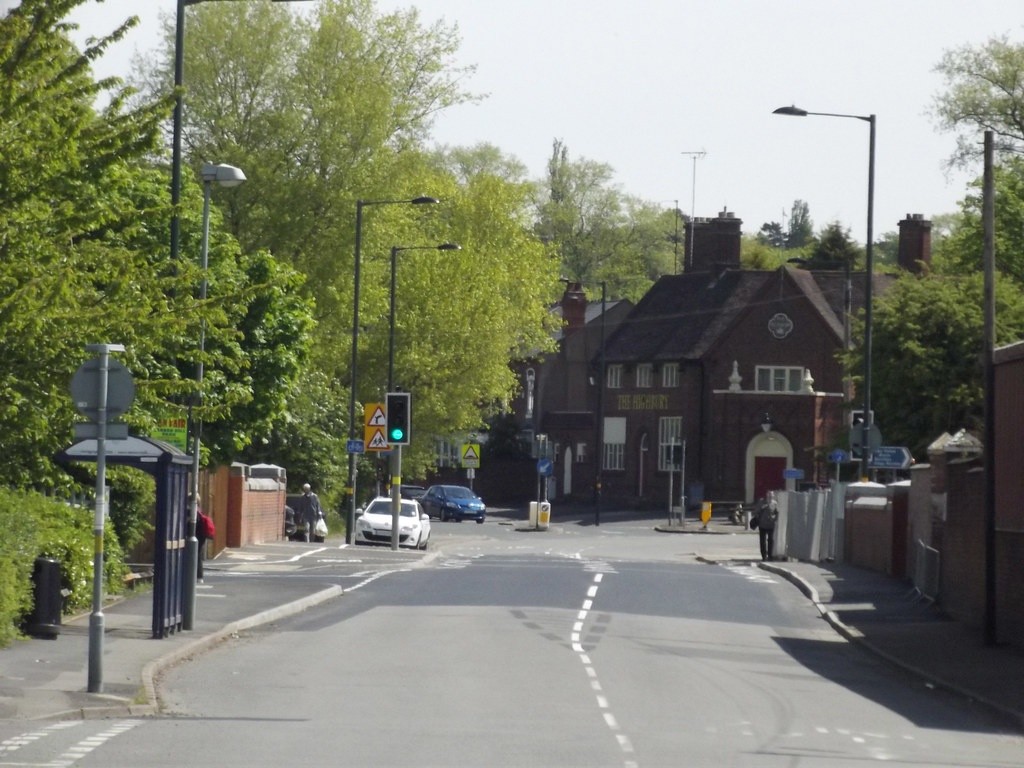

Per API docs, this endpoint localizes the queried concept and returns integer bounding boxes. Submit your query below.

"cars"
[400,484,427,504]
[420,485,486,524]
[285,506,297,535]
[354,496,431,550]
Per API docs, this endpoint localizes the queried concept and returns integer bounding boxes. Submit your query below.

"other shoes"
[196,578,203,583]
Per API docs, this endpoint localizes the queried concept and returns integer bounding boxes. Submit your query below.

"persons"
[296,483,324,542]
[754,490,779,562]
[187,492,207,583]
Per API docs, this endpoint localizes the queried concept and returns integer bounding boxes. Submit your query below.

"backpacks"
[196,510,215,540]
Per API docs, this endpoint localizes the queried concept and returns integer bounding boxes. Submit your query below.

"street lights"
[346,195,441,547]
[180,161,248,633]
[772,106,876,482]
[558,278,607,526]
[785,257,853,438]
[84,343,127,692]
[381,242,463,492]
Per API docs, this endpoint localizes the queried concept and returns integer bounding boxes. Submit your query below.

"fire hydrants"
[733,504,745,525]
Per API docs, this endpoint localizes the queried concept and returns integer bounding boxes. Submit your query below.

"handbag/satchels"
[314,518,328,537]
[750,517,758,529]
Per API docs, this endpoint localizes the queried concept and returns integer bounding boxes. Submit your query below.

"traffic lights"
[852,410,874,461]
[386,393,410,445]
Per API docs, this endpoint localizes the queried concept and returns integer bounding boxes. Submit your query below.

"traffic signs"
[868,446,913,469]
